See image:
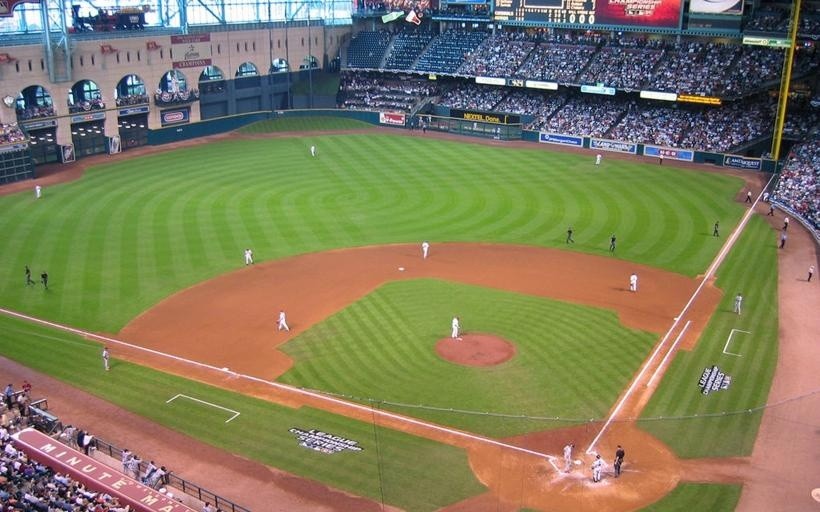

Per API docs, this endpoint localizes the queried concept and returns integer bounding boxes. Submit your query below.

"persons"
[102,345,112,374]
[565,225,576,245]
[276,308,290,334]
[628,269,639,293]
[39,268,50,291]
[0,374,228,512]
[33,181,43,199]
[767,200,777,214]
[590,452,603,484]
[731,292,743,318]
[563,442,574,474]
[422,238,430,260]
[658,154,664,166]
[335,1,820,156]
[779,232,787,251]
[594,151,603,168]
[805,263,816,283]
[613,445,625,480]
[770,156,820,238]
[609,233,617,254]
[310,143,315,157]
[24,261,35,289]
[243,246,255,267]
[713,220,720,239]
[744,188,754,204]
[451,314,460,341]
[0,86,202,144]
